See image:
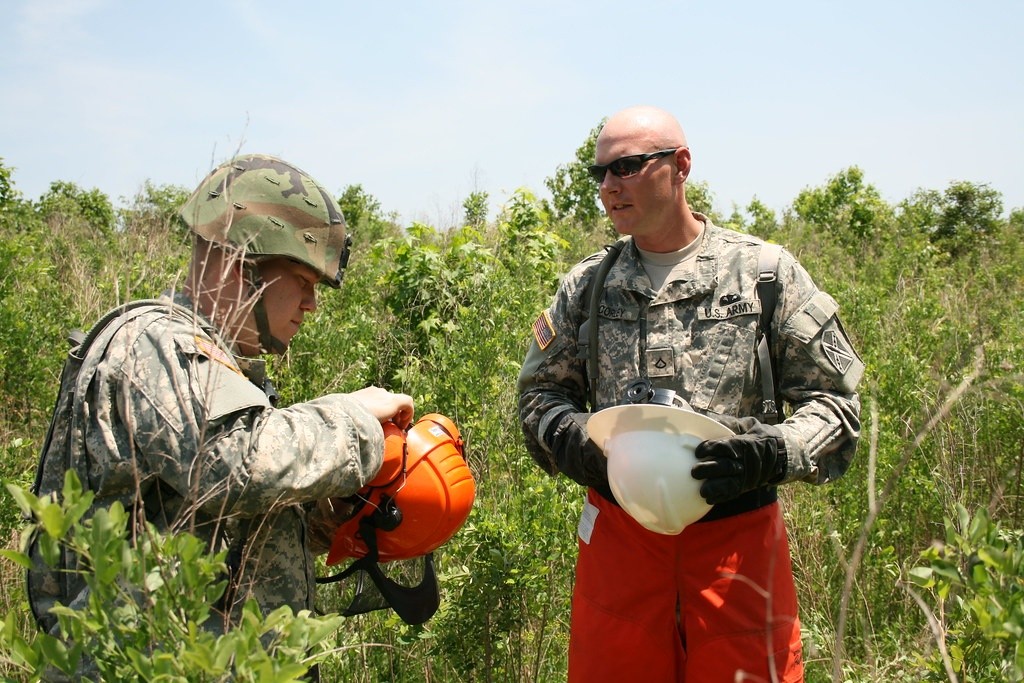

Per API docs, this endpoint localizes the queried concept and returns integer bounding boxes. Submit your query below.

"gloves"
[691,416,788,506]
[551,411,622,512]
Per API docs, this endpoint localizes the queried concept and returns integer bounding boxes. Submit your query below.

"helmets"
[179,153,353,289]
[326,413,477,567]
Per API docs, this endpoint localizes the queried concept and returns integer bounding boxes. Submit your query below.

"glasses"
[587,145,690,184]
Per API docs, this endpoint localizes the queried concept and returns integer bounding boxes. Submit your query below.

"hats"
[587,404,737,535]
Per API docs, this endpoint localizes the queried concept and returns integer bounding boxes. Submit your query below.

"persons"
[515,106,865,682]
[24,157,414,683]
